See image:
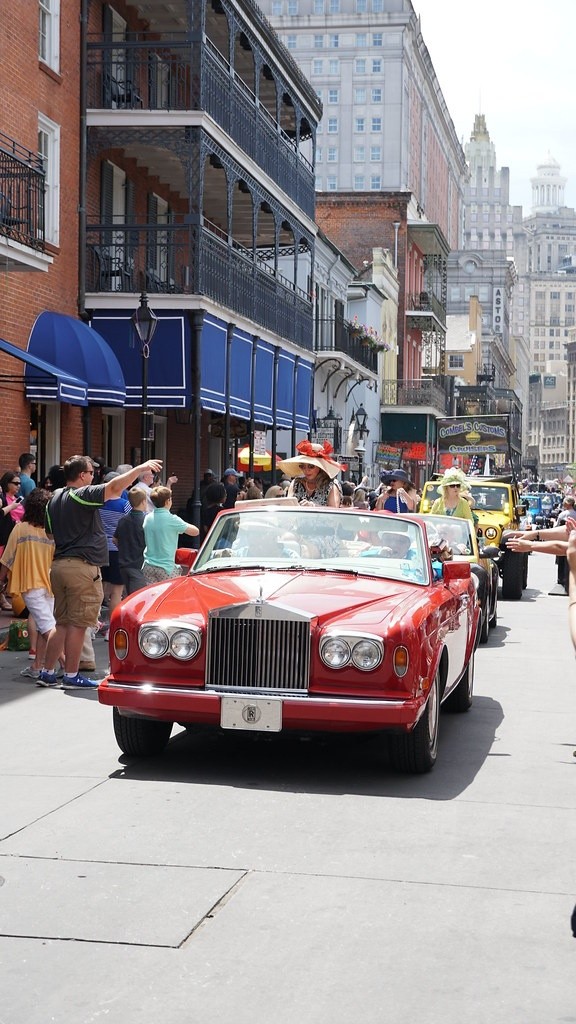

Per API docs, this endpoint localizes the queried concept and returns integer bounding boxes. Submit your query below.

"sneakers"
[60,672,99,689]
[36,672,58,686]
[20,667,40,678]
[55,668,65,677]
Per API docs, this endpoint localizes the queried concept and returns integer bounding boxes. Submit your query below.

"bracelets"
[537,529,539,541]
[569,602,576,606]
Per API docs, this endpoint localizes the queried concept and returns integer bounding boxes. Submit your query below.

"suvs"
[419,473,563,600]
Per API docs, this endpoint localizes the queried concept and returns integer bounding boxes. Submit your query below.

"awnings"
[24,310,126,407]
[0,338,88,406]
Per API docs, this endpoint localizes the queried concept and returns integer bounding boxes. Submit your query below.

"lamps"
[344,402,369,436]
[317,404,342,430]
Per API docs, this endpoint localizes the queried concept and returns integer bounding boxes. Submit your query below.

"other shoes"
[1,602,12,610]
[79,661,95,671]
[28,649,36,659]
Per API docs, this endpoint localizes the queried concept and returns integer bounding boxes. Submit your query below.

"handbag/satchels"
[7,618,31,651]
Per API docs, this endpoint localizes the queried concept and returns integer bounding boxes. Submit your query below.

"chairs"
[282,533,300,556]
[419,291,430,311]
[90,246,131,292]
[103,68,145,110]
[0,192,27,236]
[144,271,180,294]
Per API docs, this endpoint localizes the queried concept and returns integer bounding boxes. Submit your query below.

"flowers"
[348,315,391,354]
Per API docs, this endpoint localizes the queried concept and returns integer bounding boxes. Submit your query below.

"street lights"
[354,403,367,485]
[128,291,158,464]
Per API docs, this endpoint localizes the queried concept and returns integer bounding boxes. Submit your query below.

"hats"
[379,468,412,486]
[225,468,242,477]
[238,515,284,536]
[204,469,215,476]
[377,528,412,544]
[276,439,347,479]
[85,456,100,467]
[441,467,469,486]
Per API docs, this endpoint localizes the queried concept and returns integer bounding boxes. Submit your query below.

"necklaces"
[306,481,315,491]
[396,487,407,513]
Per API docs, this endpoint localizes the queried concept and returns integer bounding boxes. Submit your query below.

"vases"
[370,347,380,354]
[360,338,368,349]
[351,333,359,339]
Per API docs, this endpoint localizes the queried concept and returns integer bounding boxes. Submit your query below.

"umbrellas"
[237,444,284,472]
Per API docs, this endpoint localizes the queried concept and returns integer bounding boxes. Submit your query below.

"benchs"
[338,540,368,555]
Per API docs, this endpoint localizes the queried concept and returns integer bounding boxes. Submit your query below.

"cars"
[97,507,483,774]
[397,513,500,643]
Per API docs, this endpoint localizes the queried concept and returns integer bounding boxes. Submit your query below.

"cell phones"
[15,496,24,503]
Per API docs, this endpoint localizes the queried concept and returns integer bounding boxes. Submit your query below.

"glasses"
[449,484,460,488]
[299,464,314,469]
[84,471,93,475]
[9,481,21,486]
[32,462,37,465]
[392,480,396,482]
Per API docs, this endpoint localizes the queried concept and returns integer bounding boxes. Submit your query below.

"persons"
[187,440,503,584]
[505,478,576,758]
[0,453,201,689]
